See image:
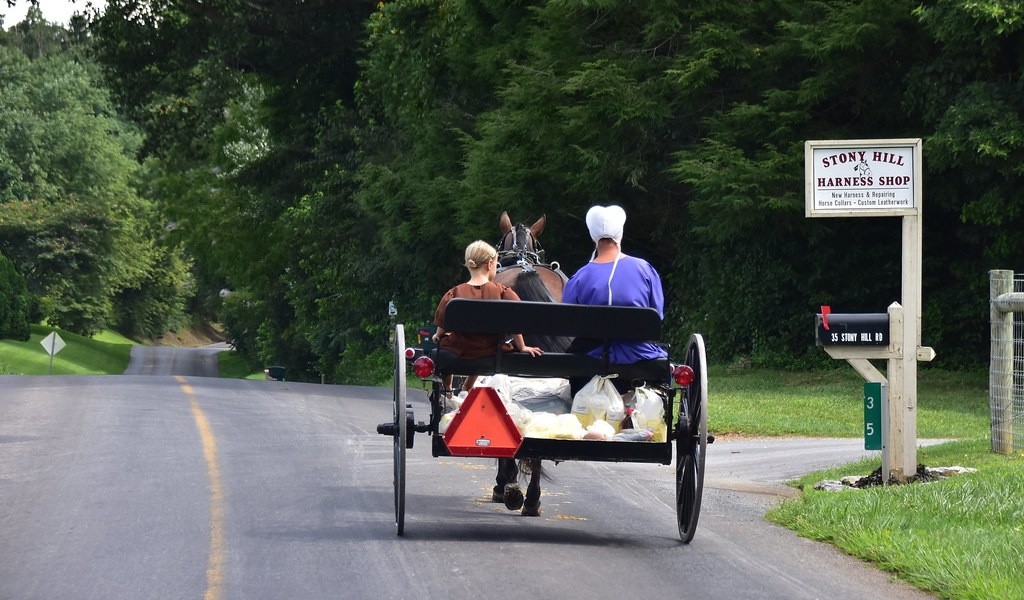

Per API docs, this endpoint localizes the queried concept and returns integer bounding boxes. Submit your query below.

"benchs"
[429,297,671,388]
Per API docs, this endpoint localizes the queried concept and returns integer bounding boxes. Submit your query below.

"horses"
[491,211,570,518]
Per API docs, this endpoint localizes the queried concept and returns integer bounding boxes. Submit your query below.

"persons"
[432,239,544,399]
[561,205,668,399]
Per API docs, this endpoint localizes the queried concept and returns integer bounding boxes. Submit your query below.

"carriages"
[376,210,715,544]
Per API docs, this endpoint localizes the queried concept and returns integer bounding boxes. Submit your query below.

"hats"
[586,205,627,243]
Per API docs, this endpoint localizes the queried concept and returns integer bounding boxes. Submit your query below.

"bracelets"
[434,334,439,340]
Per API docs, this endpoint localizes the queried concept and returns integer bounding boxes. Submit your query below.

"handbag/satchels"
[571,374,624,435]
[623,381,665,432]
